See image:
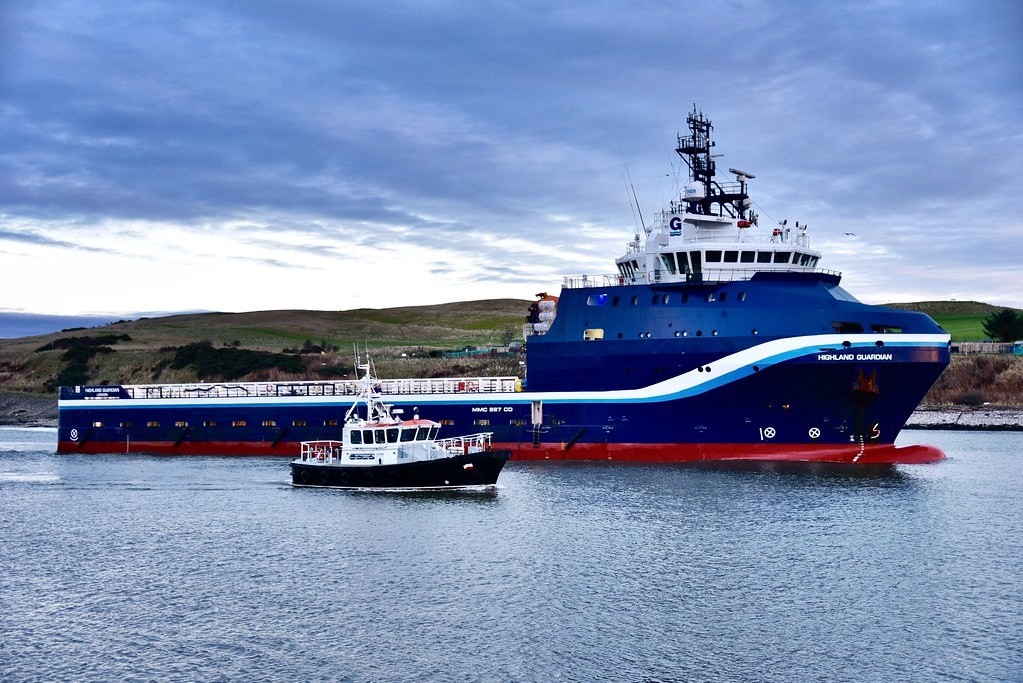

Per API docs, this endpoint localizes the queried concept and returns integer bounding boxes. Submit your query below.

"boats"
[287,338,512,493]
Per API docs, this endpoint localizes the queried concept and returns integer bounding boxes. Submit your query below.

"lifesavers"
[466,381,474,390]
[314,448,324,461]
[266,385,273,392]
[148,387,153,394]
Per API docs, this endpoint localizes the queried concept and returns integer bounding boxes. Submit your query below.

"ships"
[53,93,955,476]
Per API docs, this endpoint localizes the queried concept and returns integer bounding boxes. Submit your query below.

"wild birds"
[843,232,856,236]
